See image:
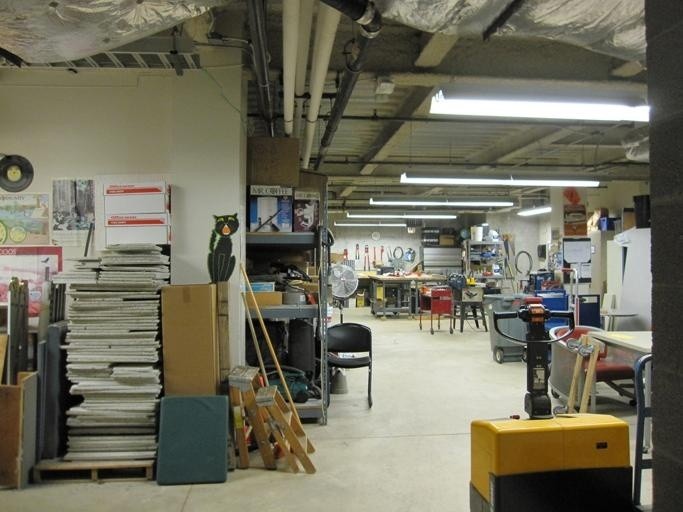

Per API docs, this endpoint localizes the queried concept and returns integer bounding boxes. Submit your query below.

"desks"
[369,274,446,321]
[587,329,654,451]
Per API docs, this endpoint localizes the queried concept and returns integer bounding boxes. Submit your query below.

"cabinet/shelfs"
[246,168,330,427]
[467,240,506,281]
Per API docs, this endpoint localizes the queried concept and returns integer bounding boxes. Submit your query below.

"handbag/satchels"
[447,271,476,289]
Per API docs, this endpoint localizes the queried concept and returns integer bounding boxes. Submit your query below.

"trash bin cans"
[484,293,534,363]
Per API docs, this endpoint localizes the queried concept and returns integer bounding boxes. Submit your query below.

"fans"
[329,263,360,358]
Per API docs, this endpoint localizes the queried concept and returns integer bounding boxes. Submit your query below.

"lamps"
[346,211,457,220]
[516,204,552,221]
[333,219,407,228]
[427,90,650,126]
[399,171,601,189]
[368,197,515,210]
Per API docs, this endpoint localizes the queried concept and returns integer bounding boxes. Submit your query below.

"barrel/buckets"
[471,227,483,240]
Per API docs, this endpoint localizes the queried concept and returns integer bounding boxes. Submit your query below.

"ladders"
[228,366,316,474]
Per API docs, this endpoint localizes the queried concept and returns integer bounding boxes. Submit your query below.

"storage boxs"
[246,136,321,233]
[439,235,455,246]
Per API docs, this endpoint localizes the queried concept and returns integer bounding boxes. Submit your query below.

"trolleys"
[415,285,454,335]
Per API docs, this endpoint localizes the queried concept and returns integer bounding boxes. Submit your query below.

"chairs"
[631,354,656,512]
[327,322,373,408]
[550,325,643,413]
[447,272,488,333]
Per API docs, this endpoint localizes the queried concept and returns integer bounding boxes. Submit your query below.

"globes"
[460,227,471,240]
